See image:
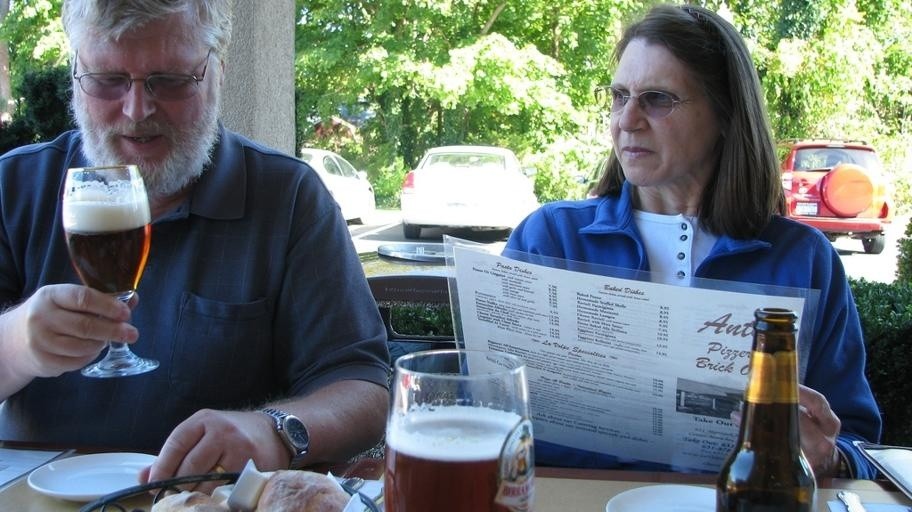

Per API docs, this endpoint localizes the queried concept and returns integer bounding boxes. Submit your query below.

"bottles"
[716,306,817,512]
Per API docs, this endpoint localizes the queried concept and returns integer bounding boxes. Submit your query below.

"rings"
[212,464,231,486]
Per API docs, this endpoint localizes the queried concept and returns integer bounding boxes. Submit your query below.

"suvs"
[770,136,898,255]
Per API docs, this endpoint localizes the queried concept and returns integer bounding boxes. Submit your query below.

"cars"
[336,99,390,129]
[300,145,378,228]
[399,144,540,241]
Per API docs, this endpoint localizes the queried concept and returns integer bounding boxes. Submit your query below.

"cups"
[382,349,536,511]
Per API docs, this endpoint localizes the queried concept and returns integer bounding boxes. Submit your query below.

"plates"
[605,484,720,511]
[26,452,165,506]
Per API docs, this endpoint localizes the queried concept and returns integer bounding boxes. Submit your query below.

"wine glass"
[61,165,162,379]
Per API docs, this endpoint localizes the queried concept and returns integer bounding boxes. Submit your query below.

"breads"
[151,459,352,511]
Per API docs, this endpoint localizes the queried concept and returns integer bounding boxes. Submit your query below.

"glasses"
[594,86,692,117]
[73,50,211,99]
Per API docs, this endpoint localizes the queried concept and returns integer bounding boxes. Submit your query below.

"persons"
[456,2,882,481]
[1,0,392,504]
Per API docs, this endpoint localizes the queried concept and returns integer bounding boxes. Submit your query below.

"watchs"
[256,407,311,470]
